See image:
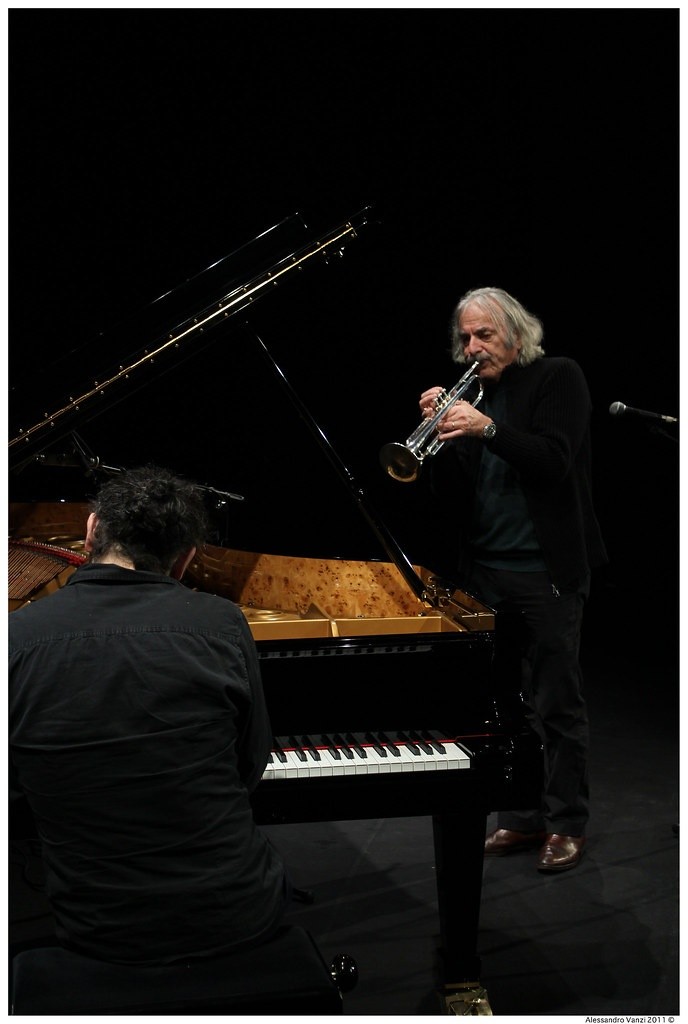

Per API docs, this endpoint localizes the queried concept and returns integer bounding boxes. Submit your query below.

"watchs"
[481,417,498,437]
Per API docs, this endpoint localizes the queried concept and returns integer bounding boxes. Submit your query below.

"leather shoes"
[536,832,585,873]
[484,827,546,855]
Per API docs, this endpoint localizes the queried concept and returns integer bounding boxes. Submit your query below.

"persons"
[9,464,350,1015]
[417,285,594,869]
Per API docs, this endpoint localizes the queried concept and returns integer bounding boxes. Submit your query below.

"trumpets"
[378,357,487,483]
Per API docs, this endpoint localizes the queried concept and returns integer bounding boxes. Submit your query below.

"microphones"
[608,401,679,425]
[39,454,104,470]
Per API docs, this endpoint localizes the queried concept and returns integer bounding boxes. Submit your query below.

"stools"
[14,921,357,1015]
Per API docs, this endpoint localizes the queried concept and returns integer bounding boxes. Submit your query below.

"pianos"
[10,199,495,1014]
